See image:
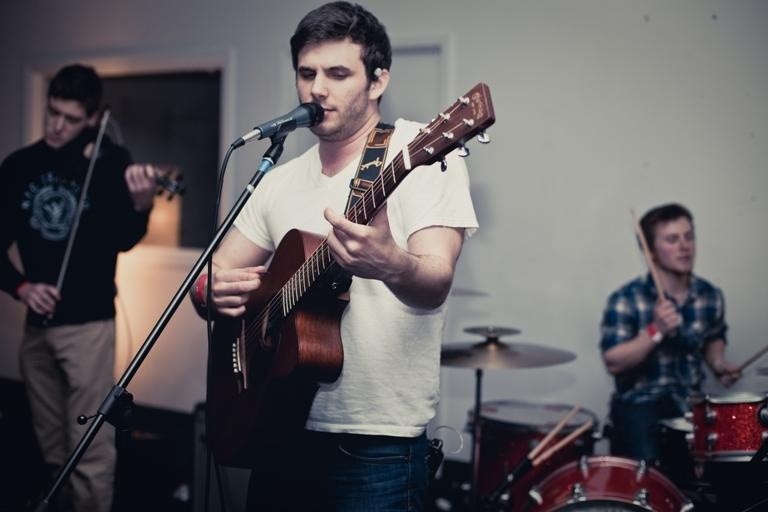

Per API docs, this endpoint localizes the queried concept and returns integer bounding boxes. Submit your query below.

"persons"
[189,1,479,511]
[0,63,159,511]
[600,203,743,467]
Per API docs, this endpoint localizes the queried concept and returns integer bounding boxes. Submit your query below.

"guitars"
[205,81,496,467]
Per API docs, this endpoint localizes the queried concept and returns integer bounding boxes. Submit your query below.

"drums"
[520,455,694,511]
[687,395,768,463]
[468,399,598,511]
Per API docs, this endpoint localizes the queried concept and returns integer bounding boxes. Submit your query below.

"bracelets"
[195,274,208,306]
[647,321,662,344]
[17,279,27,293]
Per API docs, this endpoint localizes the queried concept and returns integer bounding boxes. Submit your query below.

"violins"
[70,128,184,200]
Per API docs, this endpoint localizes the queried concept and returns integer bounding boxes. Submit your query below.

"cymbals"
[440,344,576,370]
[466,327,523,338]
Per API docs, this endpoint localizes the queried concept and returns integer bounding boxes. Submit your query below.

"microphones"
[231,102,324,149]
